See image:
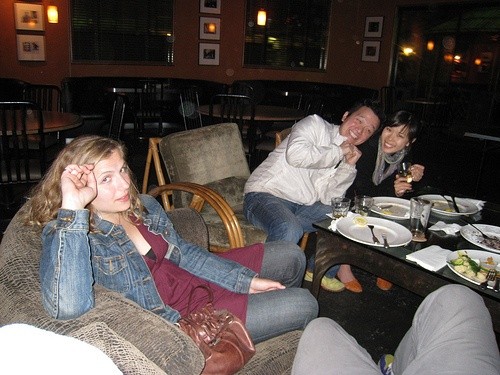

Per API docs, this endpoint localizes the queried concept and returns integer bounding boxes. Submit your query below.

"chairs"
[0,80,453,251]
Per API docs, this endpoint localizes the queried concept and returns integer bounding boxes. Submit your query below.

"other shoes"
[303,270,346,293]
[376,276,393,291]
[335,272,364,293]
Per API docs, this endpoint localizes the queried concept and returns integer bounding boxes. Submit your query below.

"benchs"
[233,80,379,110]
[61,77,216,123]
[0,78,32,110]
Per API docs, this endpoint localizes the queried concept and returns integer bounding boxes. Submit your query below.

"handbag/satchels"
[174,283,256,375]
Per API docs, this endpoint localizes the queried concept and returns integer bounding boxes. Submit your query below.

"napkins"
[326,211,360,219]
[428,221,461,235]
[406,245,453,272]
[465,198,486,210]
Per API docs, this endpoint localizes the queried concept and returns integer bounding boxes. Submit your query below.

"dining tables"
[311,187,500,333]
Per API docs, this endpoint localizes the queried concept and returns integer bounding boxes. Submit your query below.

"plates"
[370,197,412,220]
[446,249,500,290]
[335,215,412,248]
[419,195,478,216]
[460,224,500,253]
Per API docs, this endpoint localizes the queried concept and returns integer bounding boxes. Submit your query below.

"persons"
[288,279,499,375]
[334,107,437,294]
[242,99,386,295]
[36,134,321,345]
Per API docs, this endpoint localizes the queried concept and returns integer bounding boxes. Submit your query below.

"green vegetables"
[448,251,490,275]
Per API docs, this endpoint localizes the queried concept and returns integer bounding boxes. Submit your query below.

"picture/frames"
[360,40,381,63]
[199,0,222,15]
[363,16,385,38]
[199,16,221,41]
[198,42,220,66]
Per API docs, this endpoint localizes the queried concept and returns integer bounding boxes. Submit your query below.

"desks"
[0,110,85,160]
[406,98,447,119]
[197,104,304,137]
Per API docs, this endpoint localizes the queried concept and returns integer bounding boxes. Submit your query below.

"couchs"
[0,193,302,375]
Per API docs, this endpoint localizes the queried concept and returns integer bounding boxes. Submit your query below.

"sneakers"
[378,352,394,375]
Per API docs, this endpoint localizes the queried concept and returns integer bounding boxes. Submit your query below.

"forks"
[367,225,380,244]
[479,269,497,290]
[469,223,495,240]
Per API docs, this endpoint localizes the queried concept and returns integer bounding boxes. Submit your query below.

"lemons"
[384,211,393,214]
[354,216,367,226]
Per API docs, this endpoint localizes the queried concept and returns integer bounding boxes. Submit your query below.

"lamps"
[47,0,59,23]
[257,0,267,26]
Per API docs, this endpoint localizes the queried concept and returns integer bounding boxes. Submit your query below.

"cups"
[397,162,415,193]
[409,197,431,238]
[330,196,351,218]
[354,195,374,216]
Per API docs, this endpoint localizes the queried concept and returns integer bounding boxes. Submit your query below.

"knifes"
[382,233,389,249]
[450,193,460,213]
